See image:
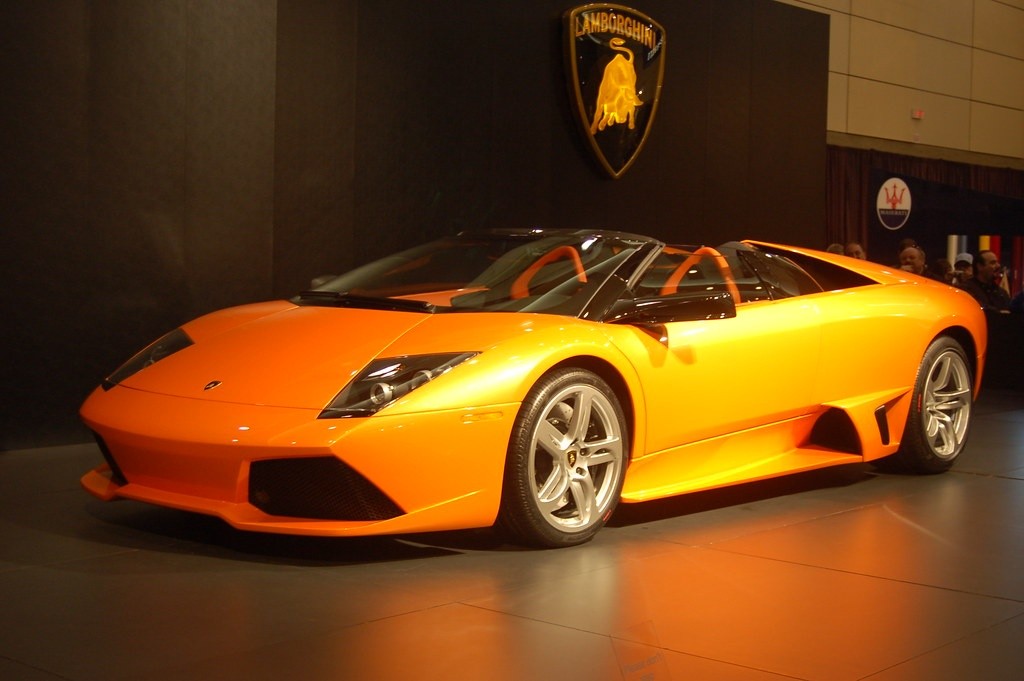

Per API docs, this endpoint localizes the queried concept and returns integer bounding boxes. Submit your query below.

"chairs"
[452,236,823,321]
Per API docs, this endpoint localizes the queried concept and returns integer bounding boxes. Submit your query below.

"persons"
[825,238,1024,329]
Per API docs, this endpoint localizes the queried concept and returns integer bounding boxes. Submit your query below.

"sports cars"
[76,225,991,551]
